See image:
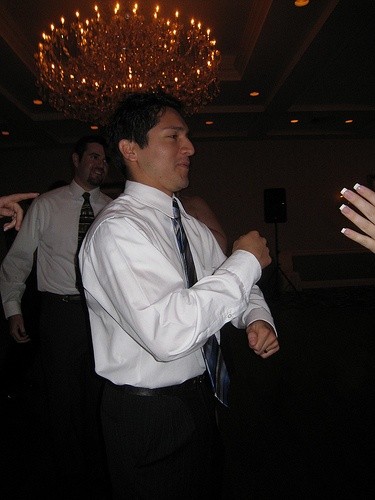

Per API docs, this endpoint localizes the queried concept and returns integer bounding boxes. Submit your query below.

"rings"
[264,350,267,353]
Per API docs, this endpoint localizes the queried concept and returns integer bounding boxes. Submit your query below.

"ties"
[170,198,233,408]
[74,192,96,296]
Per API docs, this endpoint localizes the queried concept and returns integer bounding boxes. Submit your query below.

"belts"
[109,371,208,396]
[44,292,88,303]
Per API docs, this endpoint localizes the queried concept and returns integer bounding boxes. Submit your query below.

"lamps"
[38,1,222,124]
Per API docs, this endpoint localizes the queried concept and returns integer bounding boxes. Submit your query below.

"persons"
[339,183,375,254]
[0,132,113,500]
[79,93,279,500]
[175,191,227,255]
[0,193,39,231]
